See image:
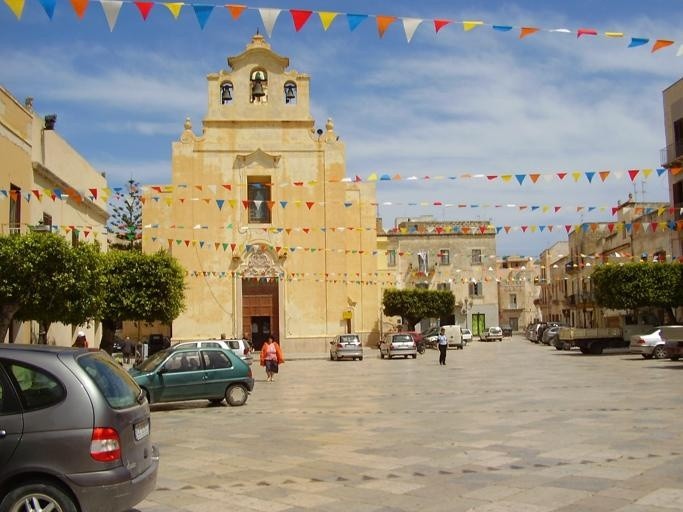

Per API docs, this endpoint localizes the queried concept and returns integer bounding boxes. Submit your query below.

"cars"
[166,341,231,366]
[500,324,513,337]
[127,347,254,407]
[629,325,683,359]
[378,328,474,354]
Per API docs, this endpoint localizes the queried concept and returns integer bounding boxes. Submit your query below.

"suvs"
[0,344,160,512]
[329,334,363,361]
[525,322,581,350]
[379,333,417,360]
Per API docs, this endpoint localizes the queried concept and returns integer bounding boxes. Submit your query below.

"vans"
[480,327,503,341]
[193,339,253,366]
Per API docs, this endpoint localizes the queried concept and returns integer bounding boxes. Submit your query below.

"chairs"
[177,357,188,371]
[189,359,198,371]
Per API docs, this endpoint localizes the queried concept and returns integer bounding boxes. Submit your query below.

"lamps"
[40,114,59,133]
[316,128,323,141]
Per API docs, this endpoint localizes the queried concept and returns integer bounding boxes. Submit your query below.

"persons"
[120,337,135,364]
[221,332,226,340]
[437,327,447,366]
[242,332,253,351]
[72,331,88,349]
[259,336,284,381]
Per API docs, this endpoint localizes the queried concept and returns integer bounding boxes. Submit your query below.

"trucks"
[559,311,660,355]
[438,323,463,351]
[659,327,682,362]
